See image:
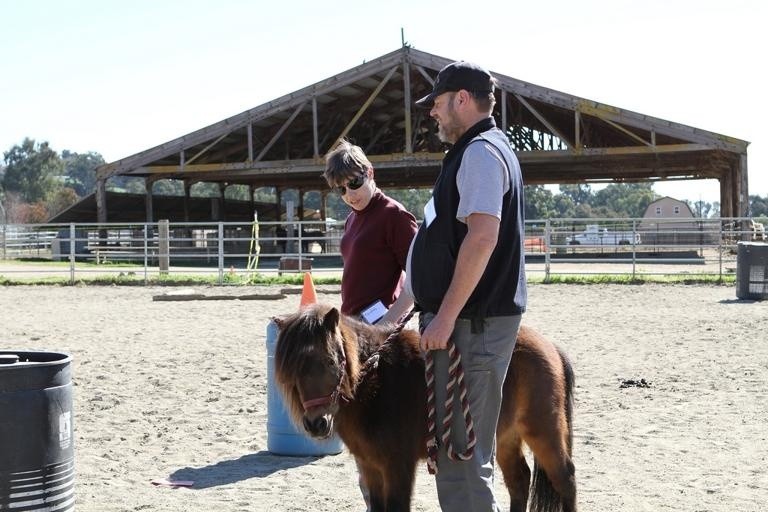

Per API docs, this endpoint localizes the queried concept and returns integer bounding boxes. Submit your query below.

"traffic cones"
[297,268,321,314]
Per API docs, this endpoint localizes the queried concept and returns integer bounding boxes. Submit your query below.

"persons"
[320,135,419,512]
[375,59,529,512]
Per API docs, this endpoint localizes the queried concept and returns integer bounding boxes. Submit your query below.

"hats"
[414,58,505,109]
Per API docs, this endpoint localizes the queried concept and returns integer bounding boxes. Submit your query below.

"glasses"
[330,172,373,199]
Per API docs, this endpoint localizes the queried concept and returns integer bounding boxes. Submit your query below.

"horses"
[266,300,580,512]
[275,227,326,255]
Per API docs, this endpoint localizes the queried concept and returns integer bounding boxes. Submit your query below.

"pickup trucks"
[565,224,642,245]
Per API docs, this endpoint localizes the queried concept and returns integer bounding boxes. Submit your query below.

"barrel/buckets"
[735,241,768,301]
[0,350,75,512]
[266,317,345,457]
